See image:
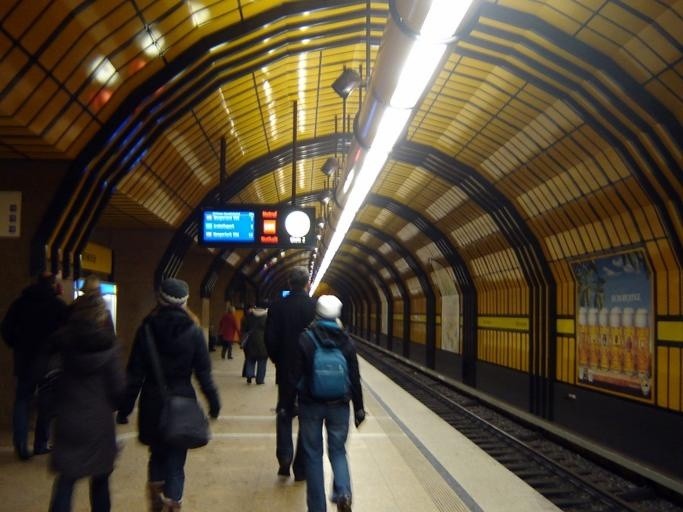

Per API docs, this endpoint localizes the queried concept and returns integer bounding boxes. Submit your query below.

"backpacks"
[307,326,350,399]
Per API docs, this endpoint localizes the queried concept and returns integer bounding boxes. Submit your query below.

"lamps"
[321,157,344,177]
[318,188,337,205]
[308,217,330,287]
[330,67,371,99]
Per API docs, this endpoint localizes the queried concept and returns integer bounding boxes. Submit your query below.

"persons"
[216,304,241,360]
[62,275,118,338]
[115,277,219,511]
[242,265,366,512]
[33,292,125,510]
[0,270,67,462]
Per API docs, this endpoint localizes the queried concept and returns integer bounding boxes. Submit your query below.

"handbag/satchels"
[159,393,210,449]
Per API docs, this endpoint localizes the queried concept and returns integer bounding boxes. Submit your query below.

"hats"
[158,278,190,306]
[315,295,343,319]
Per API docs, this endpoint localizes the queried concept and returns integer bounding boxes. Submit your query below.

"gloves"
[354,409,365,428]
